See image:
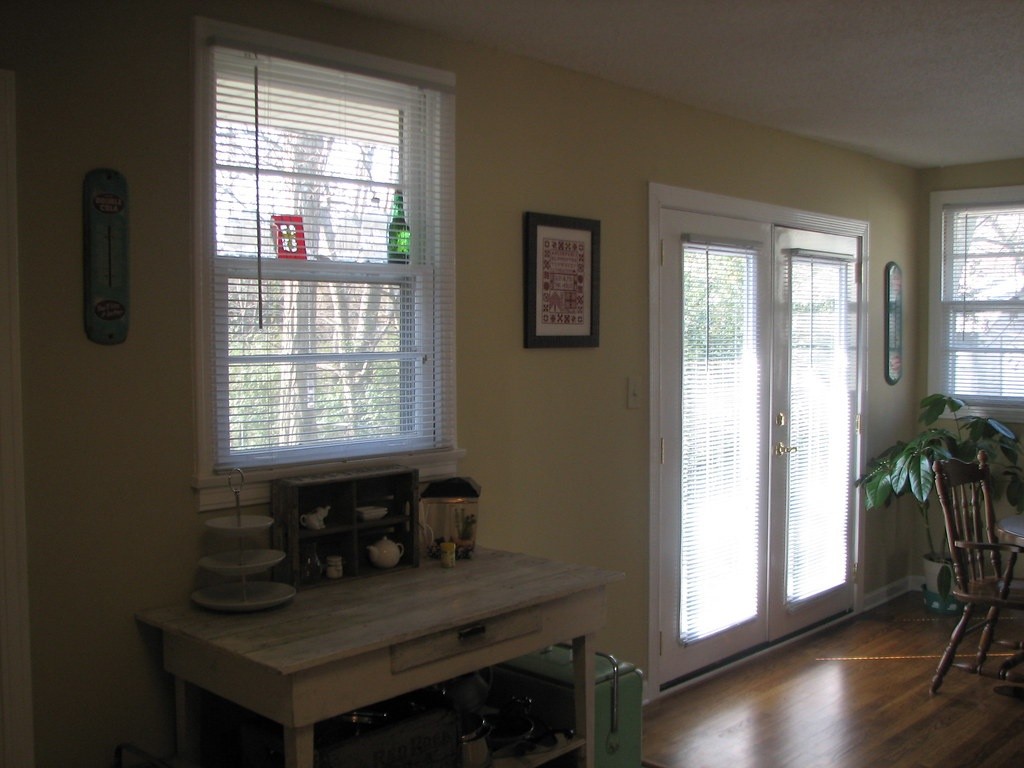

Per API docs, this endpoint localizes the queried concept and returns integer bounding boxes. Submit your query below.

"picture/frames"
[521,210,600,350]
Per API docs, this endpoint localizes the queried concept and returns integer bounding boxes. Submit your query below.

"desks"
[132,543,627,768]
[993,514,1024,682]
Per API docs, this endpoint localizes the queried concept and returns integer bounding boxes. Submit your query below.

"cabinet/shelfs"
[269,461,420,592]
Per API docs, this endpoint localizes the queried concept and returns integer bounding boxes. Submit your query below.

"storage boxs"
[238,691,464,768]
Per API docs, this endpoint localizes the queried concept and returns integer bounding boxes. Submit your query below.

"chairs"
[930,449,1024,698]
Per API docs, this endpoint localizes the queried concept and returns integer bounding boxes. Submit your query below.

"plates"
[203,514,275,538]
[198,549,286,576]
[355,505,387,521]
[191,581,297,609]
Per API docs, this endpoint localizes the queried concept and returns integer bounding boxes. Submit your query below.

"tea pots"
[367,536,404,569]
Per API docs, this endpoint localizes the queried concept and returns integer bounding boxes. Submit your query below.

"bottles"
[441,543,456,567]
[325,556,342,578]
[387,190,411,265]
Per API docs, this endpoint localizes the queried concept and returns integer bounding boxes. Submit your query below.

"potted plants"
[854,390,1024,616]
